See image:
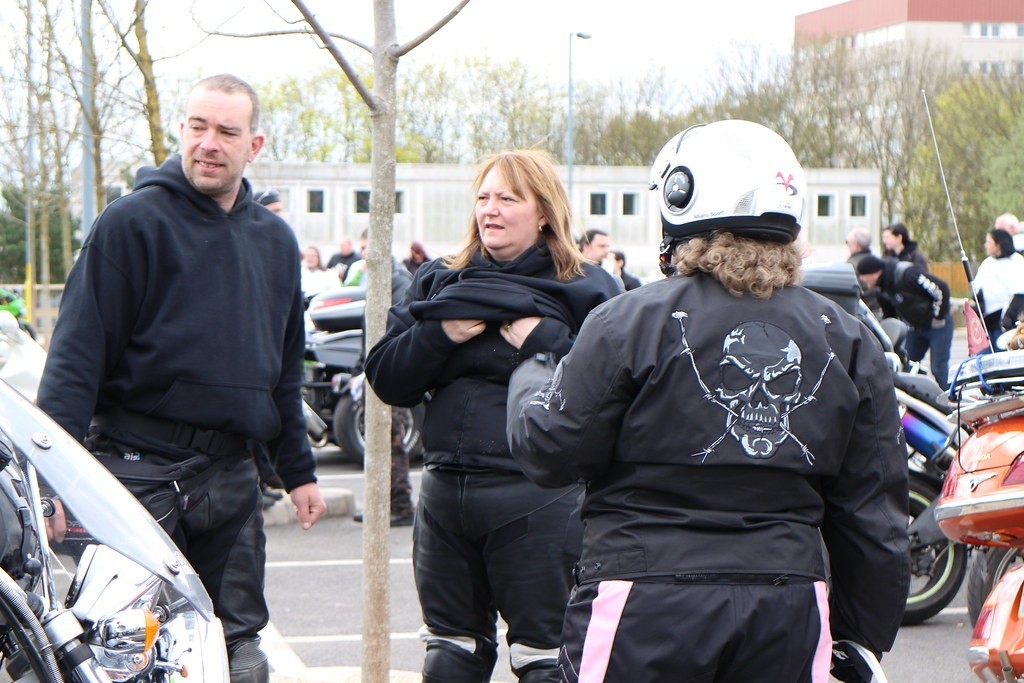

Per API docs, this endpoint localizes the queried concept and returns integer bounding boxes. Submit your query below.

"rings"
[504,322,511,329]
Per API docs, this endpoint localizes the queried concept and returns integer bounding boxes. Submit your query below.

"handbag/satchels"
[88,450,212,540]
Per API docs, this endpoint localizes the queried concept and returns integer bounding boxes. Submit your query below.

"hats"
[857,256,882,275]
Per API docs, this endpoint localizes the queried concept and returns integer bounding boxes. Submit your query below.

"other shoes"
[356,513,413,526]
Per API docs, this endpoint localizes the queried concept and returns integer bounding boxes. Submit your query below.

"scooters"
[0,289,232,683]
[885,349,1024,683]
[300,259,428,463]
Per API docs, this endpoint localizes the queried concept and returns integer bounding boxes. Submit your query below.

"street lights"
[566,30,593,215]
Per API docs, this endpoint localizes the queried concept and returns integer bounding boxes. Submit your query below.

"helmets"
[647,119,806,248]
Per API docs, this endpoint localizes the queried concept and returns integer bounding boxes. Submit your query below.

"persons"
[881,224,928,272]
[361,151,626,683]
[328,238,361,283]
[506,120,911,683]
[300,246,341,297]
[252,190,283,508]
[361,228,413,306]
[402,240,430,275]
[846,228,883,322]
[972,212,1024,392]
[36,73,326,683]
[608,250,641,292]
[580,229,625,290]
[856,256,954,385]
